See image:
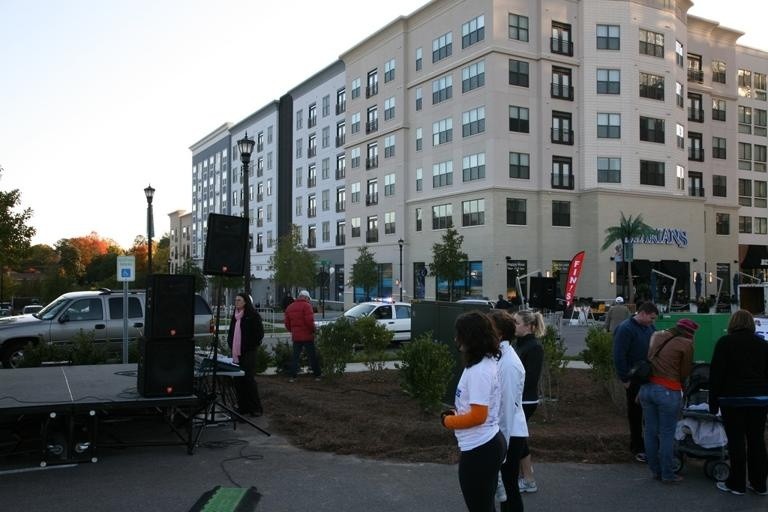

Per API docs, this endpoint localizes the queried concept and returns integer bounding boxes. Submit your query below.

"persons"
[631,301,645,318]
[512,311,546,494]
[439,309,507,511]
[379,308,389,318]
[731,295,736,304]
[639,319,701,483]
[495,294,519,313]
[757,271,765,282]
[284,290,323,383]
[248,287,295,314]
[682,386,710,436]
[612,302,657,463]
[707,308,767,495]
[227,292,264,417]
[489,311,530,511]
[697,297,707,314]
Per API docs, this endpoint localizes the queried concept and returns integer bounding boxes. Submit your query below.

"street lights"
[312,264,336,318]
[144,184,159,274]
[396,237,405,301]
[236,131,257,298]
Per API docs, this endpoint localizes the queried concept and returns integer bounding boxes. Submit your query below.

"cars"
[0,300,89,317]
[313,296,412,342]
[454,299,497,310]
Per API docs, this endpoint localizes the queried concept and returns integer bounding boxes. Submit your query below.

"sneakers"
[746,483,768,496]
[288,376,322,383]
[516,465,538,493]
[716,481,745,496]
[653,472,684,484]
[634,451,648,463]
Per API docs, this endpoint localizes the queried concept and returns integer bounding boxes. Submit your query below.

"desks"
[192,370,247,433]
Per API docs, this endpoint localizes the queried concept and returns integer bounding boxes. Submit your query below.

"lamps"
[692,269,696,284]
[708,271,712,284]
[546,270,549,279]
[609,270,615,285]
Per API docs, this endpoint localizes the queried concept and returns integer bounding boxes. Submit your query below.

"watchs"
[604,296,631,332]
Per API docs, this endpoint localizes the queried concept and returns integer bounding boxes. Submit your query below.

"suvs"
[0,289,216,369]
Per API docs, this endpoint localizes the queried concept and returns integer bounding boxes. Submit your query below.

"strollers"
[671,361,732,483]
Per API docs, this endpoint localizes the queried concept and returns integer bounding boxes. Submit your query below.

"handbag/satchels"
[628,359,653,386]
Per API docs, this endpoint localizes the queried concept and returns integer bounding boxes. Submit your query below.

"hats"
[616,296,624,303]
[299,290,312,300]
[677,318,698,331]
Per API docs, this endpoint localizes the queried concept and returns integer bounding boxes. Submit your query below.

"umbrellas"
[694,273,702,303]
[733,272,739,298]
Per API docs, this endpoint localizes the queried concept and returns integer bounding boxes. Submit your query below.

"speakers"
[204,212,249,275]
[137,341,196,397]
[144,273,196,340]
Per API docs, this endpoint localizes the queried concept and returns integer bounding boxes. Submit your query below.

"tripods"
[192,275,270,454]
